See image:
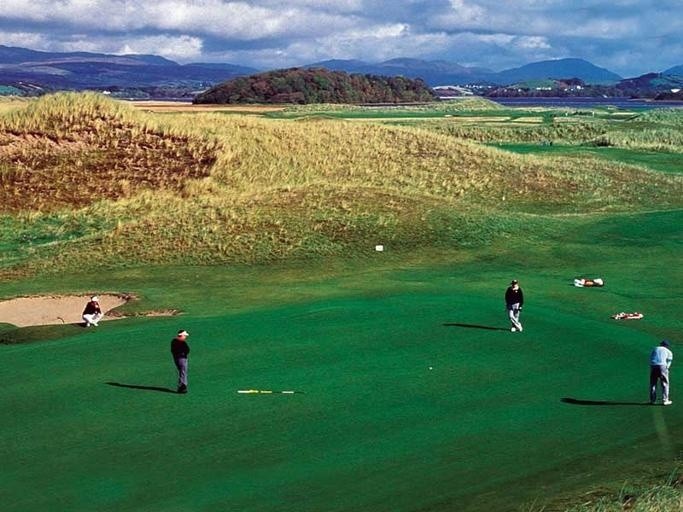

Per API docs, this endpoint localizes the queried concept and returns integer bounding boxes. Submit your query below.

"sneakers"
[85,322,90,327]
[93,323,98,327]
[510,327,515,332]
[517,323,523,332]
[662,401,671,406]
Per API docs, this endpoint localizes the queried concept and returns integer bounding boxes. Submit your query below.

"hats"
[510,280,518,284]
[91,297,98,301]
[177,331,188,336]
[660,340,669,346]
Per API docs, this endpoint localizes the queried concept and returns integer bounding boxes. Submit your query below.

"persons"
[505,277,525,334]
[80,295,104,329]
[647,340,675,406]
[169,328,192,394]
